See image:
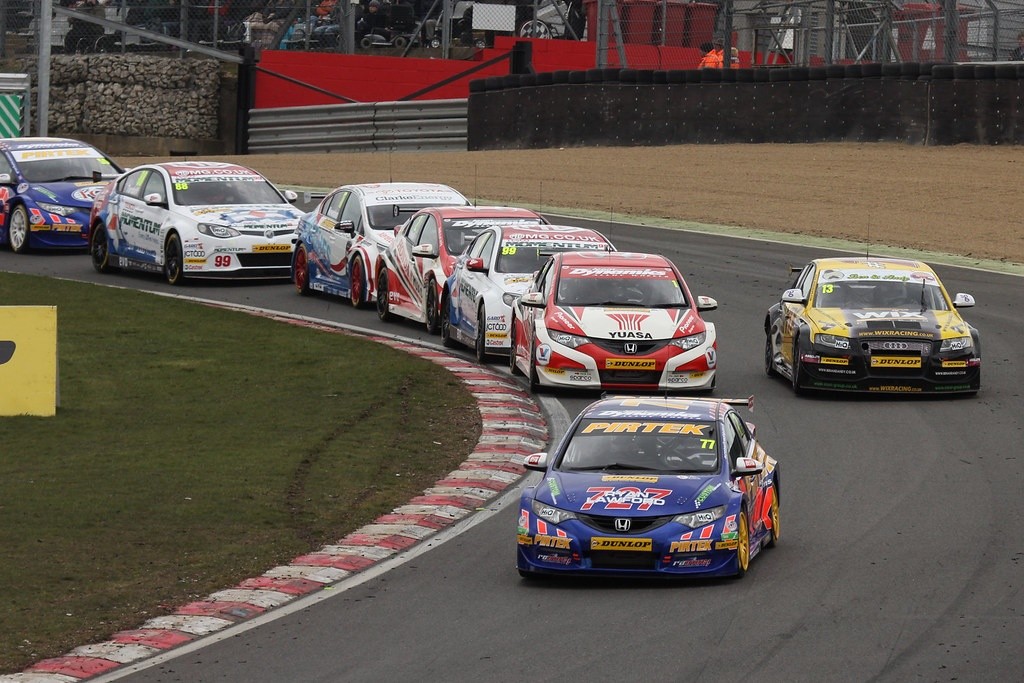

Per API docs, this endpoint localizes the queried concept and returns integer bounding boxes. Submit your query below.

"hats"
[714,38,724,47]
[369,0,380,7]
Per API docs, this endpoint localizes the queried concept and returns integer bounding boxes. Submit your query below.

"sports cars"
[440,221,622,365]
[0,136,132,253]
[87,162,318,289]
[372,205,550,333]
[288,185,480,311]
[517,391,785,585]
[762,253,986,402]
[506,248,721,400]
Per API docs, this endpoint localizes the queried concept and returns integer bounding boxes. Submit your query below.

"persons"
[354,1,390,49]
[729,47,739,70]
[712,38,725,68]
[66,0,108,54]
[309,0,337,32]
[423,14,443,46]
[697,41,721,69]
